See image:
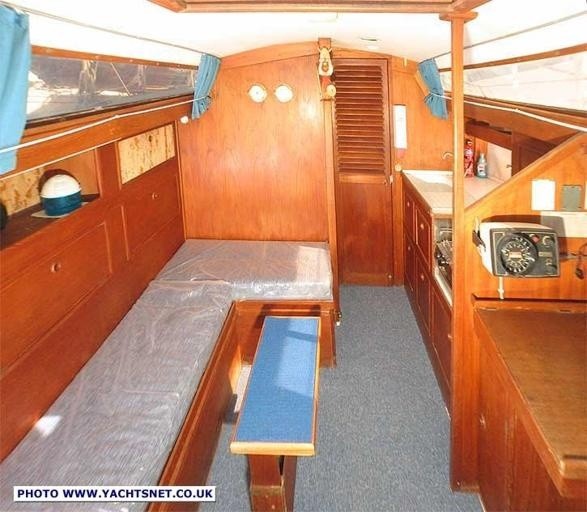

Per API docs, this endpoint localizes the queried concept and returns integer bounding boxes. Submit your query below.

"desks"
[228,314,321,510]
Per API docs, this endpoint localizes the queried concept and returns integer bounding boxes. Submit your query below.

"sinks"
[402,170,454,176]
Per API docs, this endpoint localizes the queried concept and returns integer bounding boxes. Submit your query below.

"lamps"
[402,174,429,354]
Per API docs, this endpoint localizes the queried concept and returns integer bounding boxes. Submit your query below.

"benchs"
[1,237,334,510]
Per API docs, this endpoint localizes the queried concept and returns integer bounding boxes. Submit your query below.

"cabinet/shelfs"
[116,97,184,282]
[429,277,450,416]
[0,110,114,462]
[473,307,585,510]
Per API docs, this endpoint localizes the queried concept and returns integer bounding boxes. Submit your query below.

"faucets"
[443,152,454,160]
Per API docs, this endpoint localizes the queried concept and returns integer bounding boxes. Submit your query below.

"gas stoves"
[432,239,452,291]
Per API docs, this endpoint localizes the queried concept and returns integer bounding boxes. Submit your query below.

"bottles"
[476,153,486,177]
[464,141,474,178]
